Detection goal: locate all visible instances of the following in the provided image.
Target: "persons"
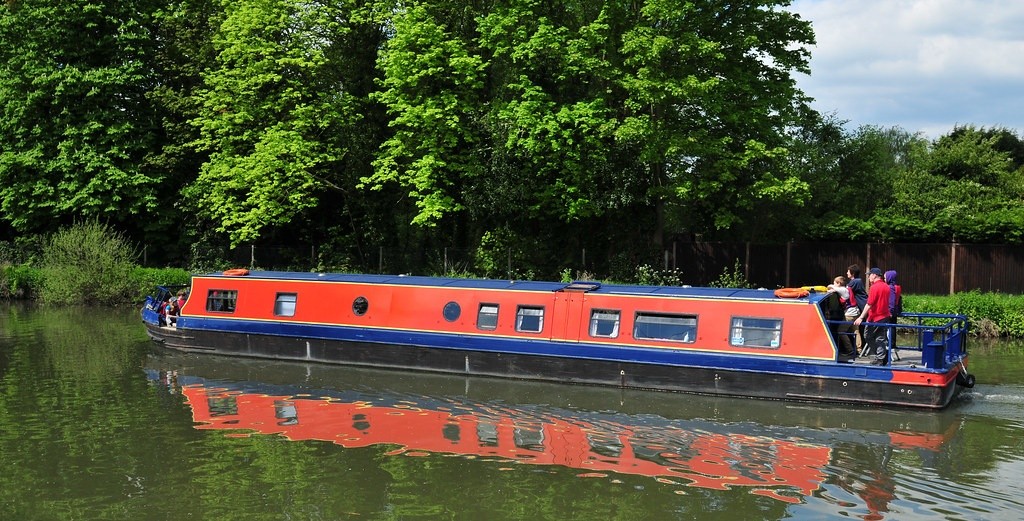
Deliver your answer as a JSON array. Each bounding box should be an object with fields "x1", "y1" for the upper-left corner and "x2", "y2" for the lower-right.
[
  {"x1": 827, "y1": 276, "x2": 862, "y2": 357},
  {"x1": 853, "y1": 268, "x2": 890, "y2": 366},
  {"x1": 884, "y1": 270, "x2": 900, "y2": 361},
  {"x1": 211, "y1": 292, "x2": 221, "y2": 311},
  {"x1": 176, "y1": 292, "x2": 186, "y2": 309},
  {"x1": 165, "y1": 297, "x2": 180, "y2": 327},
  {"x1": 846, "y1": 264, "x2": 868, "y2": 349}
]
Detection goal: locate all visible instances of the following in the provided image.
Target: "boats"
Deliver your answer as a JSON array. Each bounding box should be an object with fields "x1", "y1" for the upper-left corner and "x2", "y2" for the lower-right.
[
  {"x1": 140, "y1": 267, "x2": 977, "y2": 411},
  {"x1": 133, "y1": 342, "x2": 976, "y2": 507}
]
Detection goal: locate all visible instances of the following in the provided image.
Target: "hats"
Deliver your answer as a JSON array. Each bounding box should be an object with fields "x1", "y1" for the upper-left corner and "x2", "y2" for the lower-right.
[
  {"x1": 865, "y1": 268, "x2": 882, "y2": 276},
  {"x1": 169, "y1": 297, "x2": 177, "y2": 303}
]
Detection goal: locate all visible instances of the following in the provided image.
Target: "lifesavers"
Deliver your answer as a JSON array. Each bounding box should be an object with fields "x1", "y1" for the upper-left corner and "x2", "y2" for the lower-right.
[
  {"x1": 223, "y1": 268, "x2": 249, "y2": 276},
  {"x1": 774, "y1": 288, "x2": 809, "y2": 298}
]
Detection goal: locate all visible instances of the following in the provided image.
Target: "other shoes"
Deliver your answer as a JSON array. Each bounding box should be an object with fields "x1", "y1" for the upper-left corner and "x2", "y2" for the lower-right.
[
  {"x1": 870, "y1": 359, "x2": 886, "y2": 366},
  {"x1": 841, "y1": 351, "x2": 854, "y2": 356},
  {"x1": 856, "y1": 353, "x2": 860, "y2": 356}
]
